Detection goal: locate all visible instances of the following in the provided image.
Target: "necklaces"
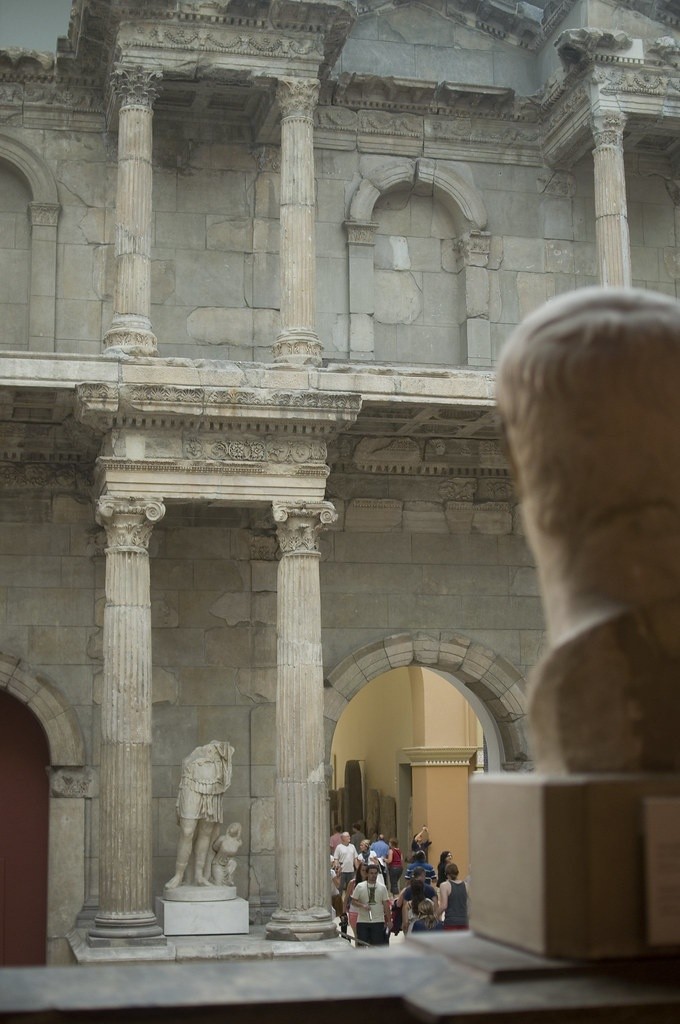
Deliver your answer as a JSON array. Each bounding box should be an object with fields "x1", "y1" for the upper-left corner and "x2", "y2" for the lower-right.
[{"x1": 367, "y1": 881, "x2": 376, "y2": 899}]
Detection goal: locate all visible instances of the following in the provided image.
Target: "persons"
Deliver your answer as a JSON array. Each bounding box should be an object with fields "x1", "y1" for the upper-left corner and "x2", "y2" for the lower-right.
[
  {"x1": 165, "y1": 740, "x2": 234, "y2": 890},
  {"x1": 329, "y1": 822, "x2": 471, "y2": 947},
  {"x1": 208, "y1": 822, "x2": 243, "y2": 887}
]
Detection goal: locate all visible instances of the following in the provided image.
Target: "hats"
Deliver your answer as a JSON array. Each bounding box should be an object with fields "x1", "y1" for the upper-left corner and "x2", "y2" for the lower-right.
[{"x1": 362, "y1": 840, "x2": 370, "y2": 847}]
